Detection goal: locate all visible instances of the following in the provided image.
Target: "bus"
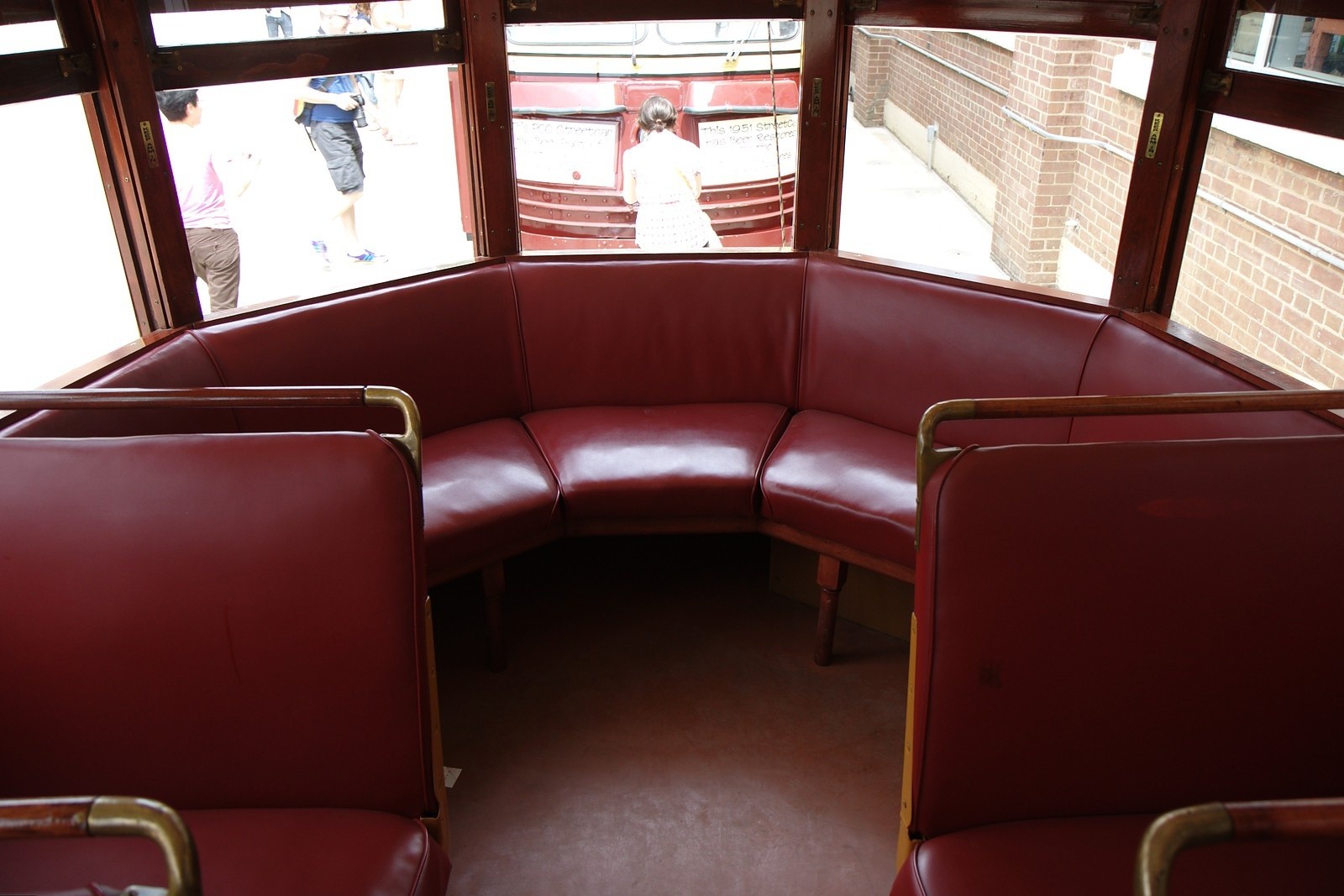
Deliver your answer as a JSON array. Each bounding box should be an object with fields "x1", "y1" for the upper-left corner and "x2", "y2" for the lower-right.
[{"x1": 449, "y1": 17, "x2": 803, "y2": 255}]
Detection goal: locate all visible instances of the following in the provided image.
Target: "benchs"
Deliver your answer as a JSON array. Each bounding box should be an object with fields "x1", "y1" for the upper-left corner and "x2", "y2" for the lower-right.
[{"x1": 0, "y1": 251, "x2": 1344, "y2": 896}]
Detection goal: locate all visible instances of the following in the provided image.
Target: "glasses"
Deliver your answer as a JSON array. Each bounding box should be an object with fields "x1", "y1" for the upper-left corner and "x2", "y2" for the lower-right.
[{"x1": 331, "y1": 13, "x2": 350, "y2": 21}]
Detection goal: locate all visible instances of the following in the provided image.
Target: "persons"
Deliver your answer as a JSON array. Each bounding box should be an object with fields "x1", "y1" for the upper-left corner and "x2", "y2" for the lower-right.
[
  {"x1": 623, "y1": 95, "x2": 711, "y2": 250},
  {"x1": 265, "y1": 7, "x2": 293, "y2": 39},
  {"x1": 350, "y1": 2, "x2": 412, "y2": 141},
  {"x1": 160, "y1": 90, "x2": 257, "y2": 312},
  {"x1": 282, "y1": 6, "x2": 388, "y2": 268}
]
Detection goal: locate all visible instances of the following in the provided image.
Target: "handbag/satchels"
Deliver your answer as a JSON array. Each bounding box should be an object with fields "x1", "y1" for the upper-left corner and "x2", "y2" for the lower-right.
[
  {"x1": 294, "y1": 98, "x2": 314, "y2": 124},
  {"x1": 703, "y1": 212, "x2": 722, "y2": 247}
]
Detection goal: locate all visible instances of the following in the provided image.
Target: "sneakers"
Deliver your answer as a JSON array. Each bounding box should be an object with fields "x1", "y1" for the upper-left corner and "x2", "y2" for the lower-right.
[
  {"x1": 309, "y1": 240, "x2": 335, "y2": 272},
  {"x1": 347, "y1": 250, "x2": 388, "y2": 264}
]
